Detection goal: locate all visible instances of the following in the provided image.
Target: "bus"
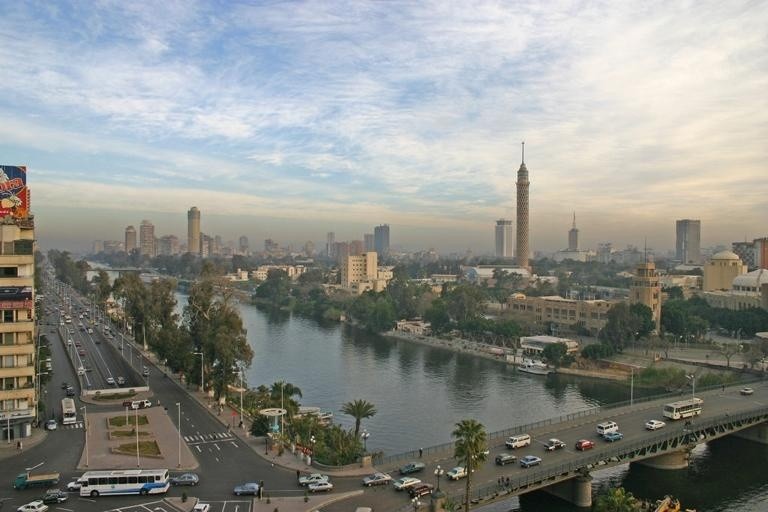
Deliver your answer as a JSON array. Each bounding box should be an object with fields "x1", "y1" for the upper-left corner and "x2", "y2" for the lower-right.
[
  {"x1": 663, "y1": 398, "x2": 704, "y2": 421},
  {"x1": 80, "y1": 469, "x2": 170, "y2": 498},
  {"x1": 61, "y1": 398, "x2": 77, "y2": 424}
]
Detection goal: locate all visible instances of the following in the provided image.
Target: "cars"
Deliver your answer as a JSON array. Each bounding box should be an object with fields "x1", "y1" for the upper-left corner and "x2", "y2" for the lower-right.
[
  {"x1": 740, "y1": 388, "x2": 754, "y2": 395},
  {"x1": 46, "y1": 420, "x2": 58, "y2": 431},
  {"x1": 17, "y1": 478, "x2": 81, "y2": 512},
  {"x1": 42, "y1": 281, "x2": 150, "y2": 396},
  {"x1": 299, "y1": 474, "x2": 333, "y2": 492},
  {"x1": 192, "y1": 503, "x2": 210, "y2": 512},
  {"x1": 234, "y1": 483, "x2": 260, "y2": 496},
  {"x1": 646, "y1": 420, "x2": 666, "y2": 431},
  {"x1": 172, "y1": 474, "x2": 199, "y2": 486},
  {"x1": 363, "y1": 421, "x2": 623, "y2": 498}
]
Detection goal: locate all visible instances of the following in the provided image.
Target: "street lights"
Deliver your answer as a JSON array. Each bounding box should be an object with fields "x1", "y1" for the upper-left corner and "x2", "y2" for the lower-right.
[
  {"x1": 631, "y1": 366, "x2": 641, "y2": 406},
  {"x1": 80, "y1": 402, "x2": 181, "y2": 467},
  {"x1": 685, "y1": 374, "x2": 694, "y2": 425},
  {"x1": 232, "y1": 371, "x2": 243, "y2": 421},
  {"x1": 412, "y1": 497, "x2": 421, "y2": 512},
  {"x1": 282, "y1": 382, "x2": 286, "y2": 436},
  {"x1": 361, "y1": 428, "x2": 370, "y2": 450},
  {"x1": 194, "y1": 353, "x2": 204, "y2": 392},
  {"x1": 310, "y1": 436, "x2": 315, "y2": 455}
]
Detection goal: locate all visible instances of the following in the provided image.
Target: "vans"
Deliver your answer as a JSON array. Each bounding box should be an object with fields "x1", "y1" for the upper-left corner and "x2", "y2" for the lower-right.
[
  {"x1": 131, "y1": 400, "x2": 152, "y2": 410},
  {"x1": 356, "y1": 507, "x2": 372, "y2": 512}
]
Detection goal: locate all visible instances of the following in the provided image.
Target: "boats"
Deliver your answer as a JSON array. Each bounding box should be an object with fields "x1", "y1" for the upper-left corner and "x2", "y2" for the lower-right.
[{"x1": 517, "y1": 363, "x2": 556, "y2": 375}]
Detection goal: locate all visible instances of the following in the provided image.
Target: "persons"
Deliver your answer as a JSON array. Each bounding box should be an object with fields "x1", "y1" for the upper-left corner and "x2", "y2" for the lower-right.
[
  {"x1": 296, "y1": 468, "x2": 300, "y2": 480},
  {"x1": 497, "y1": 475, "x2": 510, "y2": 487},
  {"x1": 419, "y1": 447, "x2": 424, "y2": 457}
]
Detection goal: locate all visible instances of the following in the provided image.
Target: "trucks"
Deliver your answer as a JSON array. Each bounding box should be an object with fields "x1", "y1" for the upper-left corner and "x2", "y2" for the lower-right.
[{"x1": 13, "y1": 472, "x2": 60, "y2": 491}]
